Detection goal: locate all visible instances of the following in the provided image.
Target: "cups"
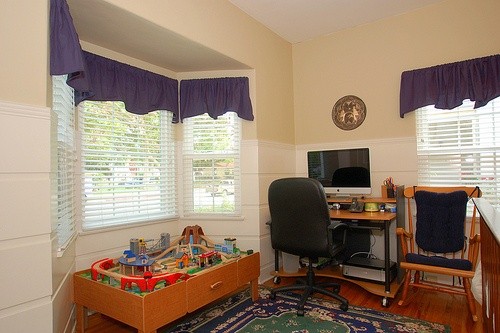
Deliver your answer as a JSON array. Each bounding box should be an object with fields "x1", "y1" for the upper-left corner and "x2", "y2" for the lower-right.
[{"x1": 387, "y1": 188, "x2": 394, "y2": 199}]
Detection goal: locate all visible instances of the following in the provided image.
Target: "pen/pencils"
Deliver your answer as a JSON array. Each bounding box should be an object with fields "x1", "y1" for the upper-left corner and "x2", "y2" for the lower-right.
[{"x1": 384, "y1": 177, "x2": 393, "y2": 189}]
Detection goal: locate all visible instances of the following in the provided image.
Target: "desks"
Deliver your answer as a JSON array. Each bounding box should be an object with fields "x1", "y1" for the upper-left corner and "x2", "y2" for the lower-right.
[
  {"x1": 71, "y1": 250, "x2": 260, "y2": 333},
  {"x1": 261, "y1": 189, "x2": 409, "y2": 307}
]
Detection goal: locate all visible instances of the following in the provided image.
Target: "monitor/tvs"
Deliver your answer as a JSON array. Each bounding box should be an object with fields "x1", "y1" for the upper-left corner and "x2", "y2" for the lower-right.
[{"x1": 307, "y1": 148, "x2": 372, "y2": 194}]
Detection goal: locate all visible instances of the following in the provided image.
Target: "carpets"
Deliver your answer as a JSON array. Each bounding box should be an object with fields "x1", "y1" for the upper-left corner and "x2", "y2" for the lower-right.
[{"x1": 158, "y1": 282, "x2": 452, "y2": 333}]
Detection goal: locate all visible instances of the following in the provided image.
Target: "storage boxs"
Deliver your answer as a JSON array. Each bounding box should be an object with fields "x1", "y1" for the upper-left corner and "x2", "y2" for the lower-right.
[{"x1": 343, "y1": 256, "x2": 397, "y2": 285}]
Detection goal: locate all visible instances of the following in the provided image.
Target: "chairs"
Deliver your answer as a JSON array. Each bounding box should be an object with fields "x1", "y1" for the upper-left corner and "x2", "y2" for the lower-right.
[
  {"x1": 396, "y1": 186, "x2": 484, "y2": 323},
  {"x1": 269, "y1": 178, "x2": 349, "y2": 317}
]
